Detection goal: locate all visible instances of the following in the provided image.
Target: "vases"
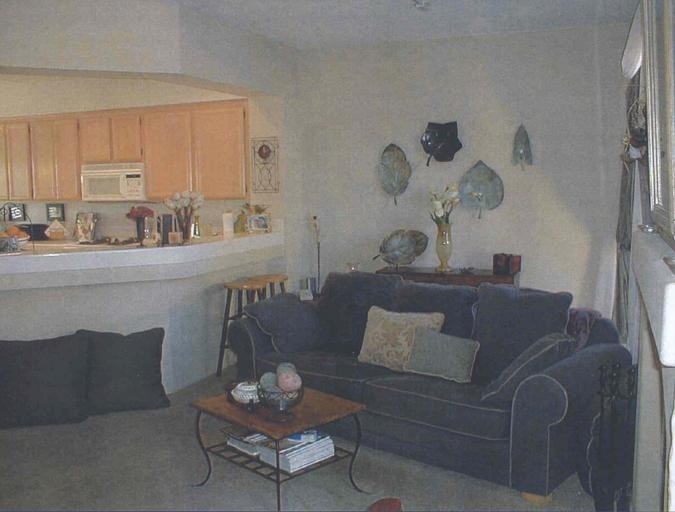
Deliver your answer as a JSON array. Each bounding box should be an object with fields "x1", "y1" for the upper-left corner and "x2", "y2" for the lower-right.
[
  {"x1": 136, "y1": 217, "x2": 145, "y2": 248},
  {"x1": 435, "y1": 221, "x2": 452, "y2": 273}
]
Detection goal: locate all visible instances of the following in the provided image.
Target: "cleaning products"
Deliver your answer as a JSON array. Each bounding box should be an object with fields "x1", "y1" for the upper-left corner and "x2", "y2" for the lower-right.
[{"x1": 193, "y1": 215, "x2": 202, "y2": 236}]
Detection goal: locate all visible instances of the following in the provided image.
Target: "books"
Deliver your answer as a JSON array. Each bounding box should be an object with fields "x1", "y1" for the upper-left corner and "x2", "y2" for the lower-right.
[{"x1": 219, "y1": 424, "x2": 336, "y2": 475}]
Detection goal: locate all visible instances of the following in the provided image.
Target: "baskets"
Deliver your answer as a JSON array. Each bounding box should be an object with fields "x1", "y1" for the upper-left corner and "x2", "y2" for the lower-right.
[{"x1": 257, "y1": 386, "x2": 305, "y2": 423}]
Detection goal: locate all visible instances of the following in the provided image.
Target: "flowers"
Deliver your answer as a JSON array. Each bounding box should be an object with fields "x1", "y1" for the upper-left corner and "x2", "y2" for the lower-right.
[
  {"x1": 420, "y1": 176, "x2": 485, "y2": 223},
  {"x1": 126, "y1": 205, "x2": 153, "y2": 219},
  {"x1": 163, "y1": 188, "x2": 203, "y2": 243}
]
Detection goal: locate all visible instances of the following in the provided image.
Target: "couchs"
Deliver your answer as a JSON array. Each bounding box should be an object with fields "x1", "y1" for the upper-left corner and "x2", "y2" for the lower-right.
[{"x1": 231, "y1": 274, "x2": 632, "y2": 495}]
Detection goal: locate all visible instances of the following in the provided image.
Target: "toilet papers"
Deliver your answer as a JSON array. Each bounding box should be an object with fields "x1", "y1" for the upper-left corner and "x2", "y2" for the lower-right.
[{"x1": 222, "y1": 213, "x2": 234, "y2": 234}]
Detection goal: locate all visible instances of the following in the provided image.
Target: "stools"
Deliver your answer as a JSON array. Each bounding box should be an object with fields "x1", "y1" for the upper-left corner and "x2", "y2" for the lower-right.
[
  {"x1": 216, "y1": 280, "x2": 267, "y2": 379},
  {"x1": 251, "y1": 263, "x2": 288, "y2": 297}
]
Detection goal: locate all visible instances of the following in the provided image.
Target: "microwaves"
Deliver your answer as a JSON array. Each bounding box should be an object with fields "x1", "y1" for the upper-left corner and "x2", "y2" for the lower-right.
[{"x1": 80, "y1": 163, "x2": 144, "y2": 202}]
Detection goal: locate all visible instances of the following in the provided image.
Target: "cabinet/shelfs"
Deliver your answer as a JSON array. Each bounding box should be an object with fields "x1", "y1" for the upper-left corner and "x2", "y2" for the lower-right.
[
  {"x1": 140, "y1": 97, "x2": 248, "y2": 202},
  {"x1": 0, "y1": 111, "x2": 33, "y2": 203},
  {"x1": 26, "y1": 112, "x2": 79, "y2": 202},
  {"x1": 73, "y1": 108, "x2": 143, "y2": 162}
]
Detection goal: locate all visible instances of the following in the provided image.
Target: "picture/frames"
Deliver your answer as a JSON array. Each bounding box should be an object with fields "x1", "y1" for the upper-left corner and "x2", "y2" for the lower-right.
[
  {"x1": 46, "y1": 203, "x2": 64, "y2": 221},
  {"x1": 8, "y1": 204, "x2": 24, "y2": 221},
  {"x1": 638, "y1": 0, "x2": 675, "y2": 251}
]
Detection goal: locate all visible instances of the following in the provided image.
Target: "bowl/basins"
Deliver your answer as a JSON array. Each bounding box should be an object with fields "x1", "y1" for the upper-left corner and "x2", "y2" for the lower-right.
[{"x1": 17, "y1": 234, "x2": 30, "y2": 248}]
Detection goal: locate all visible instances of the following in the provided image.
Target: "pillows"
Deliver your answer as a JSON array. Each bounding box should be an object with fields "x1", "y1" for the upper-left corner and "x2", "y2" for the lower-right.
[
  {"x1": 0, "y1": 330, "x2": 94, "y2": 425},
  {"x1": 354, "y1": 298, "x2": 447, "y2": 377},
  {"x1": 401, "y1": 322, "x2": 481, "y2": 380},
  {"x1": 77, "y1": 322, "x2": 174, "y2": 415},
  {"x1": 247, "y1": 294, "x2": 319, "y2": 356},
  {"x1": 480, "y1": 327, "x2": 578, "y2": 410}
]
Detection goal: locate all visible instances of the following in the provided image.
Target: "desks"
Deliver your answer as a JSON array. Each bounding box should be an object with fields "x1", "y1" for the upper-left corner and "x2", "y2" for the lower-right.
[{"x1": 373, "y1": 263, "x2": 520, "y2": 288}]
